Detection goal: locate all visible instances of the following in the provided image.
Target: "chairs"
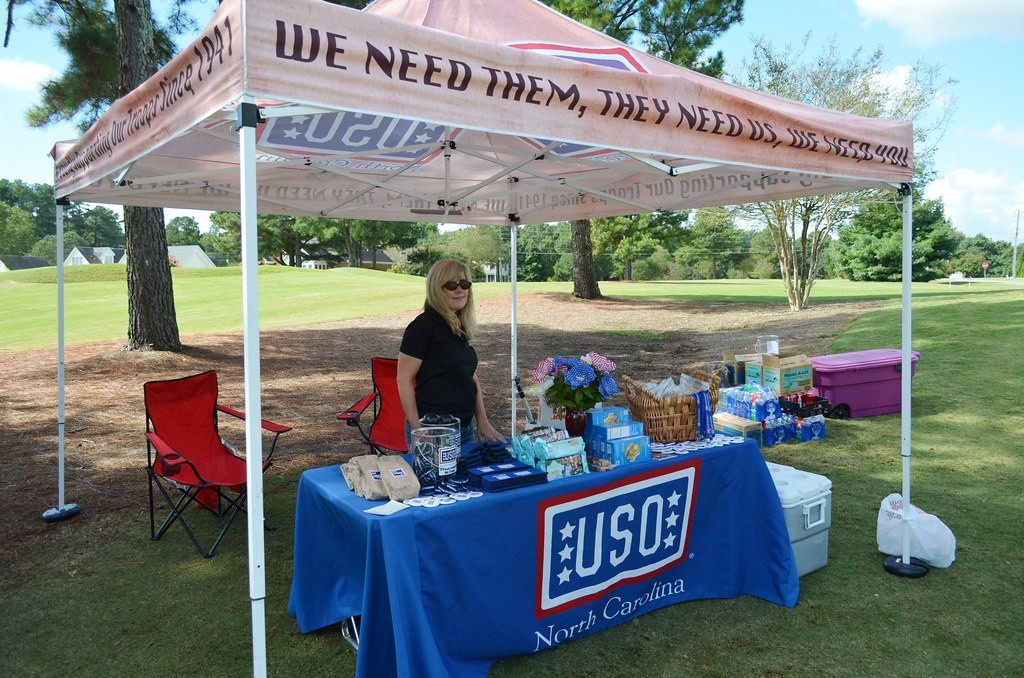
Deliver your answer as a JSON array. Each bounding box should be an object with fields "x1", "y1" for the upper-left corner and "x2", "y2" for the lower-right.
[
  {"x1": 336, "y1": 357, "x2": 415, "y2": 455},
  {"x1": 142, "y1": 369, "x2": 293, "y2": 559}
]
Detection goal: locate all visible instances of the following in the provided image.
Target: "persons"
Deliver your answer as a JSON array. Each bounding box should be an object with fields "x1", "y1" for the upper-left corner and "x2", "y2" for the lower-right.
[{"x1": 395, "y1": 258, "x2": 511, "y2": 457}]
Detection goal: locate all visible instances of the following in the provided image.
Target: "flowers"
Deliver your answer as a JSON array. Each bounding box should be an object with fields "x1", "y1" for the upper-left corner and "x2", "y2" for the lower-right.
[{"x1": 532, "y1": 351, "x2": 620, "y2": 420}]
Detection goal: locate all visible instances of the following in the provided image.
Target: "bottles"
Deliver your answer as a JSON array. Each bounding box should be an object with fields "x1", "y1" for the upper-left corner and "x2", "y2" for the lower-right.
[{"x1": 716, "y1": 384, "x2": 826, "y2": 447}]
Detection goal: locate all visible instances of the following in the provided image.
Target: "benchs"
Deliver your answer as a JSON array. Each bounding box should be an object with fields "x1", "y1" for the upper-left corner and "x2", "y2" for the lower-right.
[{"x1": 948, "y1": 271, "x2": 970, "y2": 285}]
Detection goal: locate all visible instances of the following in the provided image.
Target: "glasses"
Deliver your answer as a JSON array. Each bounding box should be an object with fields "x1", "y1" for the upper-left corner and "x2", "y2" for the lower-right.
[{"x1": 444, "y1": 279, "x2": 473, "y2": 291}]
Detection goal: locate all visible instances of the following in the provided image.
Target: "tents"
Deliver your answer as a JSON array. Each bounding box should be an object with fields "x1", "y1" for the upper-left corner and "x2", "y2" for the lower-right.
[{"x1": 39, "y1": 0, "x2": 929, "y2": 678}]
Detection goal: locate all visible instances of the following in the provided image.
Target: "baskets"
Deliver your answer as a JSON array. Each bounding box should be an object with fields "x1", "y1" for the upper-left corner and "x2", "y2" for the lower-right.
[{"x1": 623, "y1": 369, "x2": 718, "y2": 443}]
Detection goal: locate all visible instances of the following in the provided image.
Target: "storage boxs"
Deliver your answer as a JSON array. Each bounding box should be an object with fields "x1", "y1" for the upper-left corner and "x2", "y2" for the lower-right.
[
  {"x1": 537, "y1": 396, "x2": 651, "y2": 467},
  {"x1": 765, "y1": 461, "x2": 832, "y2": 581},
  {"x1": 706, "y1": 347, "x2": 922, "y2": 450}
]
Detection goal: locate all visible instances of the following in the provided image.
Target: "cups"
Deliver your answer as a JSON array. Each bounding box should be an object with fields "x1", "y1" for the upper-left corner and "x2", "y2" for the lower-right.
[
  {"x1": 410, "y1": 427, "x2": 457, "y2": 494},
  {"x1": 418, "y1": 417, "x2": 462, "y2": 468},
  {"x1": 754, "y1": 335, "x2": 779, "y2": 362}
]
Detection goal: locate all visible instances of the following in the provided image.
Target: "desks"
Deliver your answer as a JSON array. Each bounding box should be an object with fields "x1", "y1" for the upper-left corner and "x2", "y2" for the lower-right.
[{"x1": 284, "y1": 439, "x2": 800, "y2": 677}]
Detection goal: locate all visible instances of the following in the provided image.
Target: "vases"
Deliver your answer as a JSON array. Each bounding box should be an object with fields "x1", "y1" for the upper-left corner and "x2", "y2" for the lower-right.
[{"x1": 565, "y1": 410, "x2": 587, "y2": 438}]
